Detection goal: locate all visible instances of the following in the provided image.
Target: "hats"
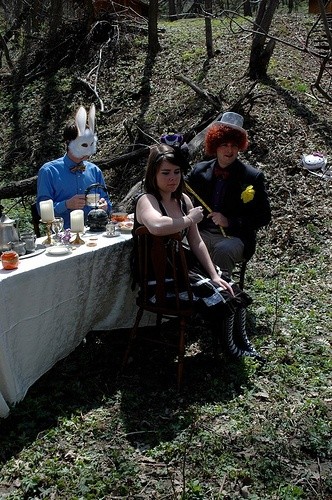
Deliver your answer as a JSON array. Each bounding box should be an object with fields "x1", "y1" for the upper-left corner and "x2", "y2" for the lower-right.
[{"x1": 211, "y1": 112, "x2": 246, "y2": 132}]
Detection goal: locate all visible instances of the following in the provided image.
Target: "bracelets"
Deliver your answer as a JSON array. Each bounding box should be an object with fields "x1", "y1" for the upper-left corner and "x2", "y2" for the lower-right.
[{"x1": 186, "y1": 216, "x2": 194, "y2": 226}]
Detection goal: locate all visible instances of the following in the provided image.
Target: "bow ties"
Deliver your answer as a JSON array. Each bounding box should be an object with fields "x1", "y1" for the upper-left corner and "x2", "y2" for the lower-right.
[
  {"x1": 214, "y1": 167, "x2": 230, "y2": 181},
  {"x1": 69, "y1": 164, "x2": 85, "y2": 175}
]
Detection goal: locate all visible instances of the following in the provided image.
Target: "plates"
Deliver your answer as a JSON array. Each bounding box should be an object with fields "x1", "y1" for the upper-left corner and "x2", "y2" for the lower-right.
[
  {"x1": 127, "y1": 213, "x2": 134, "y2": 221},
  {"x1": 103, "y1": 232, "x2": 120, "y2": 237},
  {"x1": 48, "y1": 247, "x2": 69, "y2": 255},
  {"x1": 115, "y1": 222, "x2": 134, "y2": 230}
]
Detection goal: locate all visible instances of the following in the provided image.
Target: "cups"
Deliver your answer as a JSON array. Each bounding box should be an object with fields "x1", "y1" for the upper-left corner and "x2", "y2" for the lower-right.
[
  {"x1": 106, "y1": 224, "x2": 115, "y2": 235},
  {"x1": 86, "y1": 193, "x2": 100, "y2": 209},
  {"x1": 0, "y1": 233, "x2": 36, "y2": 256}
]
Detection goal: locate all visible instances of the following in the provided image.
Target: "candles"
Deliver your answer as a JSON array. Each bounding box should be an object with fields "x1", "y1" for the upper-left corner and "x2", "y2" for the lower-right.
[
  {"x1": 70, "y1": 209, "x2": 85, "y2": 231},
  {"x1": 39, "y1": 198, "x2": 55, "y2": 222}
]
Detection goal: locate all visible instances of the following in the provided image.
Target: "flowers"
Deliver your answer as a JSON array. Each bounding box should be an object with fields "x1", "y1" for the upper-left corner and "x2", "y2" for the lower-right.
[{"x1": 240, "y1": 184, "x2": 255, "y2": 204}]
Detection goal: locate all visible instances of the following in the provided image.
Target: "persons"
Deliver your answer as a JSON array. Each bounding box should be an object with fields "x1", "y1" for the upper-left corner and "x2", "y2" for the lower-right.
[
  {"x1": 127, "y1": 143, "x2": 268, "y2": 363},
  {"x1": 183, "y1": 112, "x2": 270, "y2": 277},
  {"x1": 36, "y1": 123, "x2": 112, "y2": 232}
]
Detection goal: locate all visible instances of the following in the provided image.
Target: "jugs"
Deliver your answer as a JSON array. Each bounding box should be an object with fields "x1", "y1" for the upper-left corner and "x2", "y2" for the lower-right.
[{"x1": 0, "y1": 211, "x2": 19, "y2": 257}]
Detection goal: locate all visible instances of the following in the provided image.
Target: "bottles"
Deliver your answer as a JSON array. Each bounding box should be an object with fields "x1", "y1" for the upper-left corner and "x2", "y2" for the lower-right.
[
  {"x1": 87, "y1": 206, "x2": 108, "y2": 232},
  {"x1": 1, "y1": 251, "x2": 19, "y2": 270}
]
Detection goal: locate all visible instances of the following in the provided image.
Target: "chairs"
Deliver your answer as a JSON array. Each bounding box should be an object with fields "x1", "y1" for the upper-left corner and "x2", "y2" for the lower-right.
[{"x1": 133, "y1": 226, "x2": 218, "y2": 397}]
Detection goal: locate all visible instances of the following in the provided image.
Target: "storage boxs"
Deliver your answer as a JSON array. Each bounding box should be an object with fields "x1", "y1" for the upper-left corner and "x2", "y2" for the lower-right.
[{"x1": 111, "y1": 212, "x2": 128, "y2": 224}]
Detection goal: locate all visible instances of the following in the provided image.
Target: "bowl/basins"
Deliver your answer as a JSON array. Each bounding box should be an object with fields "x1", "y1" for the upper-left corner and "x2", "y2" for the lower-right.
[{"x1": 112, "y1": 213, "x2": 129, "y2": 221}]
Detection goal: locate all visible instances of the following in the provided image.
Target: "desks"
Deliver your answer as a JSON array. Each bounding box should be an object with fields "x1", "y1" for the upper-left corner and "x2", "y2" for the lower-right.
[{"x1": 0, "y1": 225, "x2": 189, "y2": 420}]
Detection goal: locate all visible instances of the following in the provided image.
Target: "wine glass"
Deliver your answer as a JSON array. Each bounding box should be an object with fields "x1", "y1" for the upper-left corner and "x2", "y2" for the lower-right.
[{"x1": 51, "y1": 216, "x2": 65, "y2": 244}]
